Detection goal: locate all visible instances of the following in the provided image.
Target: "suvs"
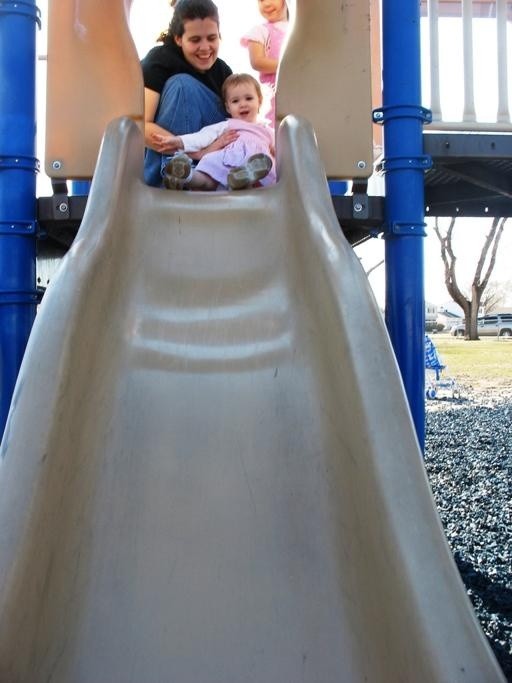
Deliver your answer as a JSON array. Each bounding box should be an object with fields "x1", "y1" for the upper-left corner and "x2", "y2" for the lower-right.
[{"x1": 450, "y1": 312, "x2": 512, "y2": 337}]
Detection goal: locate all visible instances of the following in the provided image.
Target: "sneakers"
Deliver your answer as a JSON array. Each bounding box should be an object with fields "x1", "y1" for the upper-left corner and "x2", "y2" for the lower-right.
[
  {"x1": 162, "y1": 155, "x2": 191, "y2": 190},
  {"x1": 228, "y1": 153, "x2": 272, "y2": 188}
]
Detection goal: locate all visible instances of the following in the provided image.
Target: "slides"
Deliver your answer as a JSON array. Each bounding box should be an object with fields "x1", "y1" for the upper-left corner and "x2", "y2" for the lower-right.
[{"x1": 0, "y1": 115, "x2": 507, "y2": 681}]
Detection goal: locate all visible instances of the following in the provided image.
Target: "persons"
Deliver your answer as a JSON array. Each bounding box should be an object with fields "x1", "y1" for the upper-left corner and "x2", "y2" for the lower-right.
[
  {"x1": 151, "y1": 73, "x2": 277, "y2": 190},
  {"x1": 239, "y1": 0, "x2": 291, "y2": 86},
  {"x1": 140, "y1": 1, "x2": 233, "y2": 188}
]
